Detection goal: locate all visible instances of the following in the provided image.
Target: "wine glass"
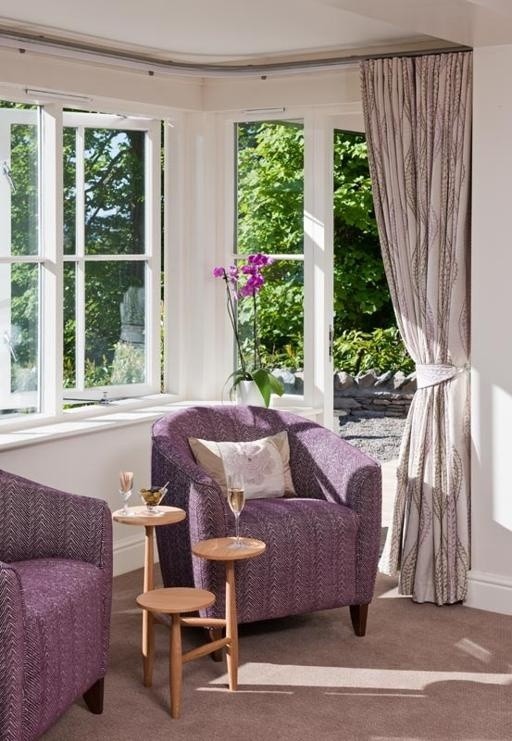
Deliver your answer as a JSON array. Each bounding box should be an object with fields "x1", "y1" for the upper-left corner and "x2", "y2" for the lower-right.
[
  {"x1": 228, "y1": 488, "x2": 245, "y2": 549},
  {"x1": 118, "y1": 472, "x2": 167, "y2": 517}
]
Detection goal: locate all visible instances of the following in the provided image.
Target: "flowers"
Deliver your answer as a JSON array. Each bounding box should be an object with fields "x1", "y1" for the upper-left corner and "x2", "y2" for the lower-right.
[{"x1": 214, "y1": 253, "x2": 286, "y2": 409}]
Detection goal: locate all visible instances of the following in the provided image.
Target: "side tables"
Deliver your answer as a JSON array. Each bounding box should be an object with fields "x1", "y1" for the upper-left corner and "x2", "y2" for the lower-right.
[{"x1": 110, "y1": 504, "x2": 266, "y2": 719}]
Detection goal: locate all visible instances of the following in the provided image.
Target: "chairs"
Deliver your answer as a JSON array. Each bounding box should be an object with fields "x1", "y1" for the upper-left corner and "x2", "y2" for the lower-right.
[
  {"x1": 151, "y1": 404, "x2": 382, "y2": 663},
  {"x1": 0, "y1": 404, "x2": 114, "y2": 740}
]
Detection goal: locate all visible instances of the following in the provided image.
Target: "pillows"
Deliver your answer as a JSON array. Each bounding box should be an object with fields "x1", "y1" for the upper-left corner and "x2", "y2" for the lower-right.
[{"x1": 187, "y1": 431, "x2": 299, "y2": 500}]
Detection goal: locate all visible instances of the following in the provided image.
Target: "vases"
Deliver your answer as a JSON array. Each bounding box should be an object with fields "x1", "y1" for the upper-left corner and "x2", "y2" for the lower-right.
[{"x1": 237, "y1": 380, "x2": 267, "y2": 407}]
